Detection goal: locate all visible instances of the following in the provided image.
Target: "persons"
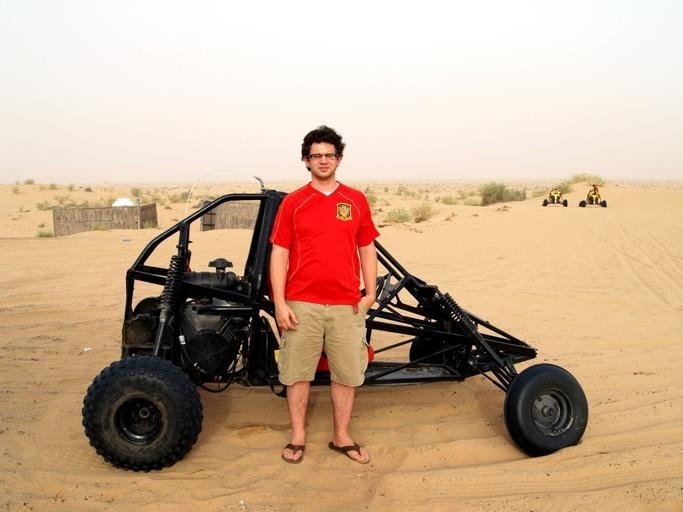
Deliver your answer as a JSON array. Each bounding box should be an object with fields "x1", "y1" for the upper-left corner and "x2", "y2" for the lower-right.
[
  {"x1": 592, "y1": 185, "x2": 598, "y2": 194},
  {"x1": 268, "y1": 126, "x2": 380, "y2": 464}
]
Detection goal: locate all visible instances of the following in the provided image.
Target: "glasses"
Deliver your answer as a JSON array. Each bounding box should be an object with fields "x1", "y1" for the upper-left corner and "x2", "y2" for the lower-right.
[{"x1": 307, "y1": 153, "x2": 339, "y2": 161}]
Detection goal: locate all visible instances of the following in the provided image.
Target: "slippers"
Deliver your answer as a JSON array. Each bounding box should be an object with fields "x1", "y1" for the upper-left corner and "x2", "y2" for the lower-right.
[
  {"x1": 327, "y1": 441, "x2": 371, "y2": 465},
  {"x1": 282, "y1": 442, "x2": 305, "y2": 464}
]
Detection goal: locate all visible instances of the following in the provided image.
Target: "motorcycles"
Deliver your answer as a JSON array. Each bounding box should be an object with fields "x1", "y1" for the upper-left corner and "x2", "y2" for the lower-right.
[
  {"x1": 579, "y1": 185, "x2": 608, "y2": 208},
  {"x1": 541, "y1": 186, "x2": 569, "y2": 207},
  {"x1": 81, "y1": 179, "x2": 588, "y2": 472}
]
